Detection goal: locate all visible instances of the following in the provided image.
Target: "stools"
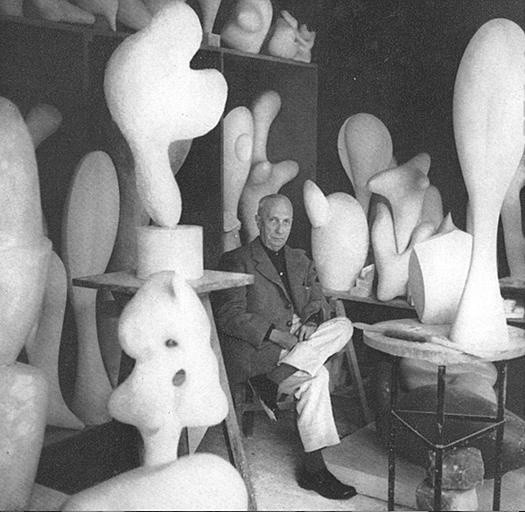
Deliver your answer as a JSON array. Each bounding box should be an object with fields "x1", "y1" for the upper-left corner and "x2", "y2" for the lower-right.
[{"x1": 234, "y1": 364, "x2": 303, "y2": 441}]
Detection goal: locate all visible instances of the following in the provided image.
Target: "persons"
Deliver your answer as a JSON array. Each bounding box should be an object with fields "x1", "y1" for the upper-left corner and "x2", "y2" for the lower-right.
[{"x1": 209, "y1": 194, "x2": 357, "y2": 500}]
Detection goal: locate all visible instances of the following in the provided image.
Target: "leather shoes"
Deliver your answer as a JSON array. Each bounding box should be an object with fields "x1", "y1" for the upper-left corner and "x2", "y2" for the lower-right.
[
  {"x1": 247, "y1": 373, "x2": 278, "y2": 422},
  {"x1": 296, "y1": 467, "x2": 357, "y2": 499}
]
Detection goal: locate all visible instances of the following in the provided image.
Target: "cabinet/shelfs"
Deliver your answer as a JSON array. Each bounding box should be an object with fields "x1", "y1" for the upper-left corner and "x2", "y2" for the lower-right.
[{"x1": 1, "y1": 14, "x2": 320, "y2": 463}]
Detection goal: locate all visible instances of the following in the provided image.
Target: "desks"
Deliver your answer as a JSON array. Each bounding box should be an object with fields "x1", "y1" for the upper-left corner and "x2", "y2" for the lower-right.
[
  {"x1": 69, "y1": 263, "x2": 258, "y2": 512},
  {"x1": 358, "y1": 313, "x2": 524, "y2": 511},
  {"x1": 317, "y1": 286, "x2": 525, "y2": 437}
]
